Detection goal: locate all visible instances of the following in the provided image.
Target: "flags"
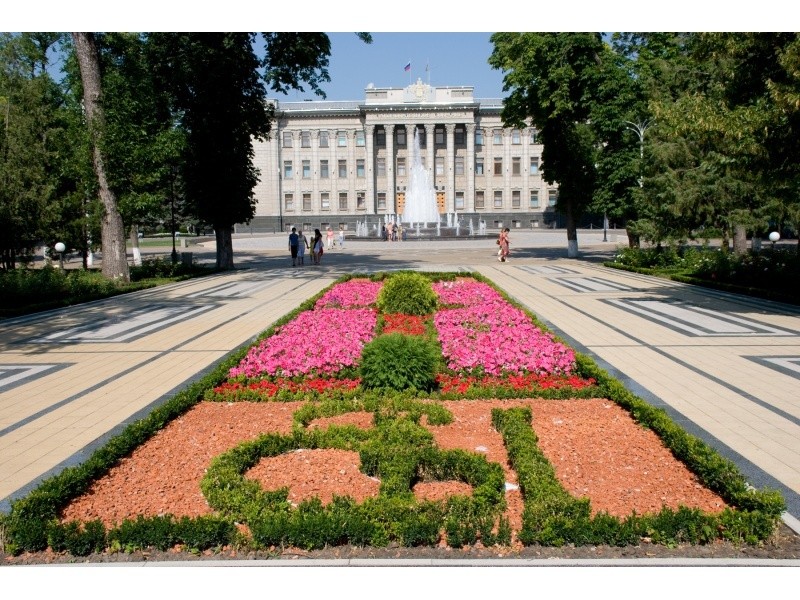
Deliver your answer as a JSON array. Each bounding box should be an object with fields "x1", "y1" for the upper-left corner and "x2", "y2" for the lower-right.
[{"x1": 404, "y1": 63, "x2": 410, "y2": 71}]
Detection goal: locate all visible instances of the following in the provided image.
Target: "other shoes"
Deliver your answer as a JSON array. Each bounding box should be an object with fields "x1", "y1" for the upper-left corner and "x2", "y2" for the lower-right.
[
  {"x1": 501, "y1": 257, "x2": 505, "y2": 262},
  {"x1": 498, "y1": 257, "x2": 501, "y2": 262}
]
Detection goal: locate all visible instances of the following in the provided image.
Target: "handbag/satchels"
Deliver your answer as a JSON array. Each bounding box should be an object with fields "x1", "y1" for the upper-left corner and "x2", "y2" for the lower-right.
[{"x1": 496, "y1": 240, "x2": 500, "y2": 245}]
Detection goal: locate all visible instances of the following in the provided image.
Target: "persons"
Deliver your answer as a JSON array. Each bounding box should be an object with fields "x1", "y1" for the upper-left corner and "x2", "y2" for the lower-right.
[
  {"x1": 382, "y1": 222, "x2": 402, "y2": 242},
  {"x1": 313, "y1": 229, "x2": 323, "y2": 265},
  {"x1": 308, "y1": 237, "x2": 315, "y2": 261},
  {"x1": 498, "y1": 227, "x2": 512, "y2": 262},
  {"x1": 326, "y1": 228, "x2": 334, "y2": 250},
  {"x1": 297, "y1": 230, "x2": 309, "y2": 265},
  {"x1": 340, "y1": 227, "x2": 344, "y2": 246},
  {"x1": 289, "y1": 227, "x2": 299, "y2": 266}
]
